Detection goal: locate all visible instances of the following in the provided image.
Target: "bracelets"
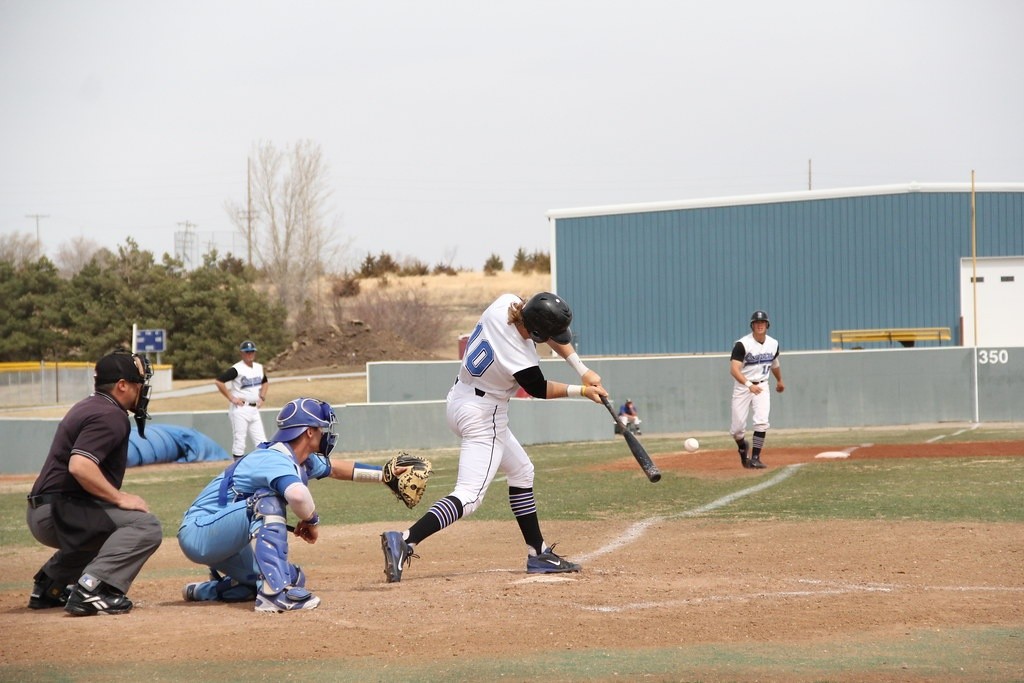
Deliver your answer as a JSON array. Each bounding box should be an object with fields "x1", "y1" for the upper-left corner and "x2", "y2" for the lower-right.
[
  {"x1": 745, "y1": 380, "x2": 753, "y2": 387},
  {"x1": 260, "y1": 396, "x2": 265, "y2": 401}
]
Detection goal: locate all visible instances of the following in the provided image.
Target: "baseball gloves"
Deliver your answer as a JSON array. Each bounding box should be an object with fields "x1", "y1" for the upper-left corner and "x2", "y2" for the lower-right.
[{"x1": 383, "y1": 451, "x2": 436, "y2": 510}]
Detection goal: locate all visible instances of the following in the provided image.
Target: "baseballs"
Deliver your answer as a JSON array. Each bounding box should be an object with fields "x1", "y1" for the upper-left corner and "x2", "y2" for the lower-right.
[{"x1": 683, "y1": 438, "x2": 699, "y2": 453}]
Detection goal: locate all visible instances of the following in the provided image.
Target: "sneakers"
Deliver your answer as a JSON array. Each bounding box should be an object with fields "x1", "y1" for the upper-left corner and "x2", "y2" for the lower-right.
[
  {"x1": 381, "y1": 529, "x2": 414, "y2": 584},
  {"x1": 65, "y1": 585, "x2": 134, "y2": 617},
  {"x1": 739, "y1": 441, "x2": 767, "y2": 468},
  {"x1": 527, "y1": 547, "x2": 582, "y2": 573},
  {"x1": 28, "y1": 583, "x2": 72, "y2": 610},
  {"x1": 181, "y1": 581, "x2": 207, "y2": 602},
  {"x1": 255, "y1": 588, "x2": 322, "y2": 613}
]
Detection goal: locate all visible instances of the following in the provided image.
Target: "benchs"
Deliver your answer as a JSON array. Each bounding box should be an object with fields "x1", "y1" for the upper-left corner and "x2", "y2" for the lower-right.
[{"x1": 831, "y1": 327, "x2": 952, "y2": 351}]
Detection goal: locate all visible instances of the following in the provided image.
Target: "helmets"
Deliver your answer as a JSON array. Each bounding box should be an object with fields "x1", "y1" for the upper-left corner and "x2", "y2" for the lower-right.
[
  {"x1": 750, "y1": 311, "x2": 770, "y2": 329},
  {"x1": 521, "y1": 291, "x2": 574, "y2": 345},
  {"x1": 268, "y1": 396, "x2": 333, "y2": 442}
]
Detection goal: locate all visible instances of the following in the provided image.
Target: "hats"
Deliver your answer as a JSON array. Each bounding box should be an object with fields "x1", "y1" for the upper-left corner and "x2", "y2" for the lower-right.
[
  {"x1": 94, "y1": 351, "x2": 149, "y2": 384},
  {"x1": 240, "y1": 340, "x2": 258, "y2": 352}
]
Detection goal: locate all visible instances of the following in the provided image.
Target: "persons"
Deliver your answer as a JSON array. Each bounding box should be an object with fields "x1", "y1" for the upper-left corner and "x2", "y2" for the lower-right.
[
  {"x1": 176, "y1": 395, "x2": 432, "y2": 613},
  {"x1": 618, "y1": 398, "x2": 642, "y2": 436},
  {"x1": 730, "y1": 310, "x2": 784, "y2": 470},
  {"x1": 380, "y1": 292, "x2": 608, "y2": 582},
  {"x1": 216, "y1": 341, "x2": 267, "y2": 462},
  {"x1": 25, "y1": 349, "x2": 161, "y2": 617}
]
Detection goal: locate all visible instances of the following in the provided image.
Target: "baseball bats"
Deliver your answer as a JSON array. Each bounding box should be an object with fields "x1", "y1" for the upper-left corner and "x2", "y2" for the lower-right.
[{"x1": 593, "y1": 384, "x2": 662, "y2": 483}]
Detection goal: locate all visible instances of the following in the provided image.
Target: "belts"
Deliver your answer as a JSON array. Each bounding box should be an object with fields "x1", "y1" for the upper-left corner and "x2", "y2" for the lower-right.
[
  {"x1": 752, "y1": 381, "x2": 765, "y2": 385},
  {"x1": 240, "y1": 402, "x2": 259, "y2": 407},
  {"x1": 28, "y1": 495, "x2": 49, "y2": 506},
  {"x1": 455, "y1": 378, "x2": 486, "y2": 397}
]
